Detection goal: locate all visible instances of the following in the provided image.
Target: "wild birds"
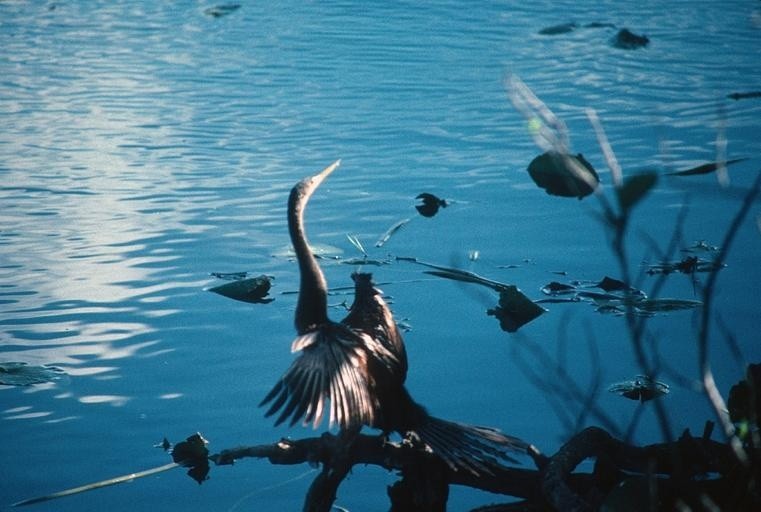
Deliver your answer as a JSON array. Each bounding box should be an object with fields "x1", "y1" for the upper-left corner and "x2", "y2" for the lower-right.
[{"x1": 256, "y1": 158, "x2": 533, "y2": 480}]
